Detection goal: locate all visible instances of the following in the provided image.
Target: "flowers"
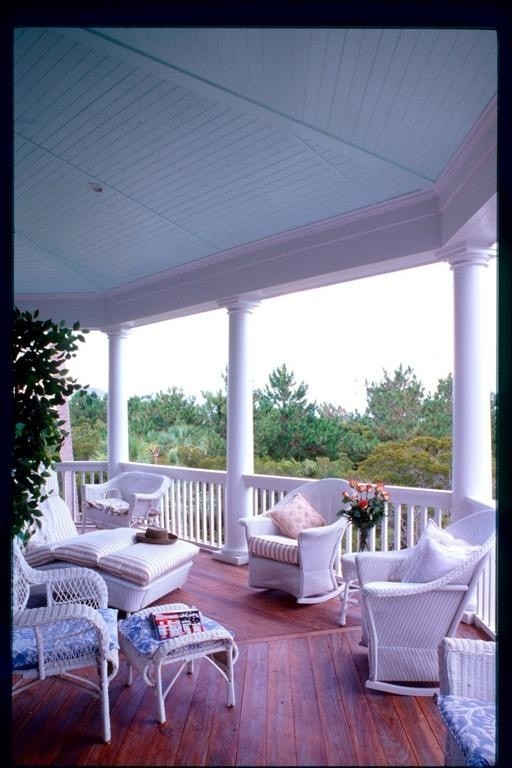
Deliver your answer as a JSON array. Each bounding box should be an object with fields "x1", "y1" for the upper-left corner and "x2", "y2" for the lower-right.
[{"x1": 342, "y1": 479, "x2": 390, "y2": 526}]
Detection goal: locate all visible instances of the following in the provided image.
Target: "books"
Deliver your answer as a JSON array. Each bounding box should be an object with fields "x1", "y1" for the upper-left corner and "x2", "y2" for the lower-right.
[{"x1": 152, "y1": 609, "x2": 206, "y2": 640}]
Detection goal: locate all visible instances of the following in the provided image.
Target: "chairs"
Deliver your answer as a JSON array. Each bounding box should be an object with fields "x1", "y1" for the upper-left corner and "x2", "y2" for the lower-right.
[
  {"x1": 75, "y1": 468, "x2": 172, "y2": 528},
  {"x1": 17, "y1": 494, "x2": 201, "y2": 614},
  {"x1": 237, "y1": 475, "x2": 348, "y2": 607},
  {"x1": 432, "y1": 630, "x2": 501, "y2": 767},
  {"x1": 9, "y1": 535, "x2": 135, "y2": 743},
  {"x1": 351, "y1": 509, "x2": 496, "y2": 698}
]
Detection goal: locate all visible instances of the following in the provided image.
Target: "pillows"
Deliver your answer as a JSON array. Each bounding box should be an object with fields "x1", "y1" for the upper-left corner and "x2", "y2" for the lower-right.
[{"x1": 266, "y1": 491, "x2": 323, "y2": 539}]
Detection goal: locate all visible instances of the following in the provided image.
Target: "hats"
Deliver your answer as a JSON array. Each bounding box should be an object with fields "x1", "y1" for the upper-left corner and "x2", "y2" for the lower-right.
[{"x1": 136, "y1": 526, "x2": 178, "y2": 544}]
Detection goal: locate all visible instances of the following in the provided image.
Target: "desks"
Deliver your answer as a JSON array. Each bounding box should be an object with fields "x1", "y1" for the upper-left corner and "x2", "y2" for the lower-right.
[
  {"x1": 340, "y1": 550, "x2": 357, "y2": 627},
  {"x1": 117, "y1": 600, "x2": 241, "y2": 726}
]
